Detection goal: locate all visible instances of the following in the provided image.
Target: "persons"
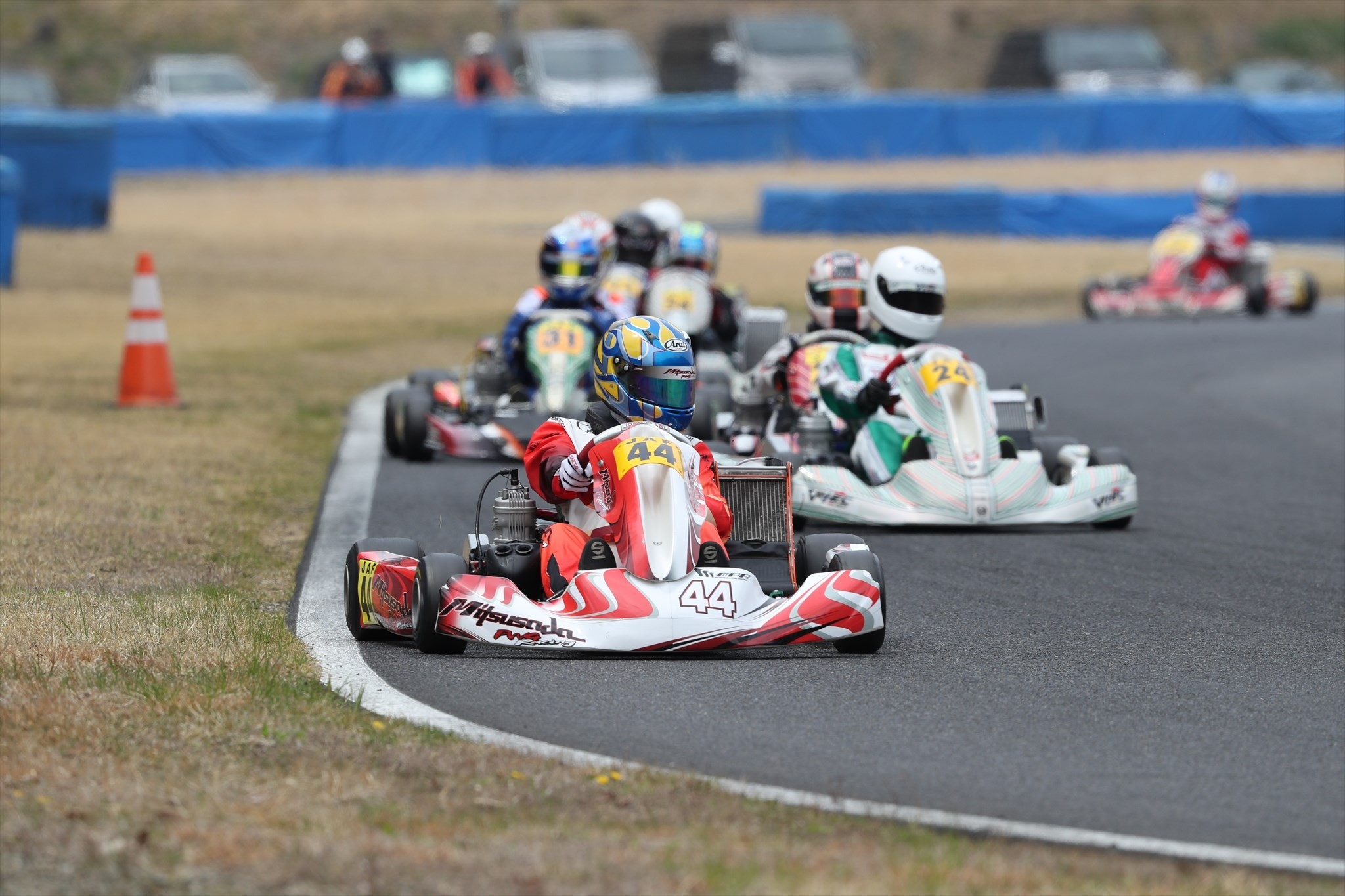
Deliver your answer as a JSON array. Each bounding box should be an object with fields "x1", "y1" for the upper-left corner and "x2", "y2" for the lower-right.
[
  {"x1": 455, "y1": 32, "x2": 515, "y2": 98},
  {"x1": 815, "y1": 246, "x2": 1020, "y2": 487},
  {"x1": 318, "y1": 38, "x2": 381, "y2": 99},
  {"x1": 501, "y1": 199, "x2": 876, "y2": 436},
  {"x1": 1169, "y1": 163, "x2": 1251, "y2": 286},
  {"x1": 523, "y1": 312, "x2": 733, "y2": 598}
]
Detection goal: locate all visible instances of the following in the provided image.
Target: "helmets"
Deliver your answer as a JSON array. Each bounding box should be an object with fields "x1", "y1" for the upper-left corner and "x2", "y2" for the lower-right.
[
  {"x1": 866, "y1": 247, "x2": 946, "y2": 342},
  {"x1": 805, "y1": 250, "x2": 872, "y2": 332},
  {"x1": 537, "y1": 225, "x2": 602, "y2": 300},
  {"x1": 637, "y1": 198, "x2": 684, "y2": 235},
  {"x1": 1194, "y1": 171, "x2": 1237, "y2": 219},
  {"x1": 641, "y1": 268, "x2": 712, "y2": 332},
  {"x1": 560, "y1": 213, "x2": 618, "y2": 281},
  {"x1": 613, "y1": 211, "x2": 660, "y2": 264},
  {"x1": 655, "y1": 221, "x2": 720, "y2": 278},
  {"x1": 594, "y1": 315, "x2": 695, "y2": 431}
]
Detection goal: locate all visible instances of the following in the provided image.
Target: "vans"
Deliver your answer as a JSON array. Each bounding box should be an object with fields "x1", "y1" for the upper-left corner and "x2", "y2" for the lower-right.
[{"x1": 657, "y1": 9, "x2": 864, "y2": 100}]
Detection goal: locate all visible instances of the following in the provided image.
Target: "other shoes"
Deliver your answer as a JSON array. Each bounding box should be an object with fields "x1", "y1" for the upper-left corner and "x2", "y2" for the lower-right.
[
  {"x1": 999, "y1": 434, "x2": 1018, "y2": 459},
  {"x1": 577, "y1": 538, "x2": 617, "y2": 571},
  {"x1": 900, "y1": 434, "x2": 930, "y2": 464},
  {"x1": 697, "y1": 541, "x2": 732, "y2": 567}
]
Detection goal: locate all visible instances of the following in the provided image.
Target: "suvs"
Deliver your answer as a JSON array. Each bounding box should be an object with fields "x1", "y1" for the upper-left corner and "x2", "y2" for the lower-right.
[
  {"x1": 501, "y1": 25, "x2": 660, "y2": 106},
  {"x1": 980, "y1": 20, "x2": 1200, "y2": 95}
]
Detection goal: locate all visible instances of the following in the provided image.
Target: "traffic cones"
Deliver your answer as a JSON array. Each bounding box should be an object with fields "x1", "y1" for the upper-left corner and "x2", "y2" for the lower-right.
[{"x1": 107, "y1": 251, "x2": 189, "y2": 411}]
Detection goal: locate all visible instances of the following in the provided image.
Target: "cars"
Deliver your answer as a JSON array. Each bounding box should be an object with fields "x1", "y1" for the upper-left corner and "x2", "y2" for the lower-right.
[
  {"x1": 305, "y1": 52, "x2": 459, "y2": 102},
  {"x1": 122, "y1": 53, "x2": 278, "y2": 112},
  {"x1": 1214, "y1": 51, "x2": 1336, "y2": 95},
  {"x1": 0, "y1": 68, "x2": 58, "y2": 108}
]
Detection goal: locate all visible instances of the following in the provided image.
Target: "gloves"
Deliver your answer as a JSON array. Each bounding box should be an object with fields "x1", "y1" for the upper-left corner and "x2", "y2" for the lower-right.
[
  {"x1": 858, "y1": 380, "x2": 894, "y2": 412},
  {"x1": 559, "y1": 453, "x2": 593, "y2": 492}
]
